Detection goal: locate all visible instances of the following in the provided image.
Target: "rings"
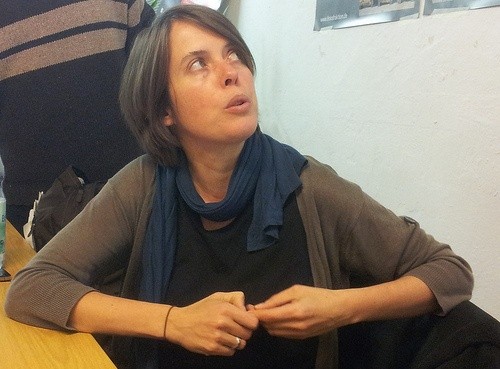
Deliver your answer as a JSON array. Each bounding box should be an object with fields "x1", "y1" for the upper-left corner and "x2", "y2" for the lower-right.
[{"x1": 230, "y1": 337, "x2": 241, "y2": 350}]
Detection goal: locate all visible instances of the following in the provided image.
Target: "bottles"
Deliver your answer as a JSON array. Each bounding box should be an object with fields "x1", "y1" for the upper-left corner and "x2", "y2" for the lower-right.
[{"x1": 0, "y1": 157, "x2": 7, "y2": 277}]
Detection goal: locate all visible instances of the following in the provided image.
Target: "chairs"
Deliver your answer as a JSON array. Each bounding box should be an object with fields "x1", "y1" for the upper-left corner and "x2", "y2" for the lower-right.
[{"x1": 343, "y1": 273, "x2": 500, "y2": 369}]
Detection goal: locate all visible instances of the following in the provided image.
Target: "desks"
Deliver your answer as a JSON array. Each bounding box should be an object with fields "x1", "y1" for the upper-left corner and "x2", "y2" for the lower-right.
[{"x1": 0, "y1": 220, "x2": 118, "y2": 369}]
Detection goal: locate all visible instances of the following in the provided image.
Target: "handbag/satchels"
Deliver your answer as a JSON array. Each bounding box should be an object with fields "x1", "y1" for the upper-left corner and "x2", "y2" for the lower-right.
[{"x1": 28, "y1": 165, "x2": 99, "y2": 251}]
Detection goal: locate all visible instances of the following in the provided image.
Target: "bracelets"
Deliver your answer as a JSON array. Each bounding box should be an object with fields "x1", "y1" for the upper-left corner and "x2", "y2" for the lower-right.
[{"x1": 163, "y1": 305, "x2": 175, "y2": 342}]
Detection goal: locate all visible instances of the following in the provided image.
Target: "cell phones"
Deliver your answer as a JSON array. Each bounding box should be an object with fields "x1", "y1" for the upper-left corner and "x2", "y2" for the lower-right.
[{"x1": 0, "y1": 268, "x2": 11, "y2": 281}]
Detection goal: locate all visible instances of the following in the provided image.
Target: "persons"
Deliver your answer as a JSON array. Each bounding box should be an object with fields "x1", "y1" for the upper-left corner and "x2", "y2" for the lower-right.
[
  {"x1": 0, "y1": 0, "x2": 159, "y2": 242},
  {"x1": 5, "y1": 4, "x2": 474, "y2": 369}
]
